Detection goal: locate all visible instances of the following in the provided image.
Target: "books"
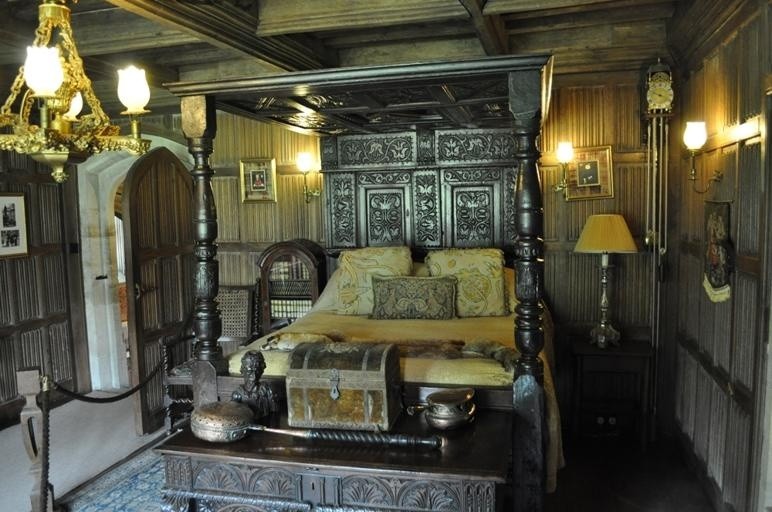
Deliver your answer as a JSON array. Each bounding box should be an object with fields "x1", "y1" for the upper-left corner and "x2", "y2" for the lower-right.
[
  {"x1": 270, "y1": 255, "x2": 311, "y2": 280},
  {"x1": 270, "y1": 299, "x2": 313, "y2": 318}
]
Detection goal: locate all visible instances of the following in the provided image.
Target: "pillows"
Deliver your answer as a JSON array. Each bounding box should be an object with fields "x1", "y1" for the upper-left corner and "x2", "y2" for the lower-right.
[
  {"x1": 425, "y1": 248, "x2": 507, "y2": 316},
  {"x1": 337, "y1": 246, "x2": 414, "y2": 316},
  {"x1": 370, "y1": 274, "x2": 458, "y2": 320}
]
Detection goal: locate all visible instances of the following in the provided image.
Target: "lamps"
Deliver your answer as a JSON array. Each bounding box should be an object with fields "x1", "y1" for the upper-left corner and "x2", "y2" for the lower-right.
[
  {"x1": 0, "y1": 0, "x2": 152, "y2": 183},
  {"x1": 296, "y1": 152, "x2": 321, "y2": 203},
  {"x1": 573, "y1": 214, "x2": 638, "y2": 349},
  {"x1": 683, "y1": 121, "x2": 723, "y2": 193},
  {"x1": 551, "y1": 142, "x2": 574, "y2": 192}
]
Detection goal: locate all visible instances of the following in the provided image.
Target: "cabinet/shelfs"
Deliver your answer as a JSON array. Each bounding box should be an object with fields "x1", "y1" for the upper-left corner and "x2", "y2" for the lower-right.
[{"x1": 257, "y1": 239, "x2": 327, "y2": 335}]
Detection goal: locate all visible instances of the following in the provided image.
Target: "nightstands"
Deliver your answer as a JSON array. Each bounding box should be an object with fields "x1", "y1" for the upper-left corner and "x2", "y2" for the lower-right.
[{"x1": 568, "y1": 335, "x2": 652, "y2": 454}]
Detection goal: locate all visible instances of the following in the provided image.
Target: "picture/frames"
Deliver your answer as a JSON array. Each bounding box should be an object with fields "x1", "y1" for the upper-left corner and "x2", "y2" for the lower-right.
[
  {"x1": 564, "y1": 145, "x2": 615, "y2": 202},
  {"x1": 0, "y1": 191, "x2": 31, "y2": 261},
  {"x1": 239, "y1": 157, "x2": 278, "y2": 203}
]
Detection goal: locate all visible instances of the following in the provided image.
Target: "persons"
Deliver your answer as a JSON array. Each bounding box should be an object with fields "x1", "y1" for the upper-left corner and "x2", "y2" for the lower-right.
[
  {"x1": 254, "y1": 175, "x2": 264, "y2": 186},
  {"x1": 232, "y1": 349, "x2": 280, "y2": 419}
]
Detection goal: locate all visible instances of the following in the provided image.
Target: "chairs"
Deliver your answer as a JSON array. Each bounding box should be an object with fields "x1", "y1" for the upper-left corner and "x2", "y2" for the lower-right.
[{"x1": 159, "y1": 278, "x2": 261, "y2": 437}]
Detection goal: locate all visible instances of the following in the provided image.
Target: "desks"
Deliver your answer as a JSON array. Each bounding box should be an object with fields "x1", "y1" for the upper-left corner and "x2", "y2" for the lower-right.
[{"x1": 153, "y1": 398, "x2": 515, "y2": 512}]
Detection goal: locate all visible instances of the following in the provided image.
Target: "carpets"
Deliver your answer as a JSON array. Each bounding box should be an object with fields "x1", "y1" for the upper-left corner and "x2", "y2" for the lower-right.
[{"x1": 53, "y1": 419, "x2": 190, "y2": 512}]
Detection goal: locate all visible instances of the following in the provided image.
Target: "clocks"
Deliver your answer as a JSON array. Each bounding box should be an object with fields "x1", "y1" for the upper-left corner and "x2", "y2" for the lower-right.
[{"x1": 640, "y1": 57, "x2": 675, "y2": 122}]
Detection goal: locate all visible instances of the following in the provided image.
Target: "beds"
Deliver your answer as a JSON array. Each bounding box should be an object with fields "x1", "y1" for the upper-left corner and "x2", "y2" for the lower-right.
[{"x1": 162, "y1": 46, "x2": 567, "y2": 512}]
